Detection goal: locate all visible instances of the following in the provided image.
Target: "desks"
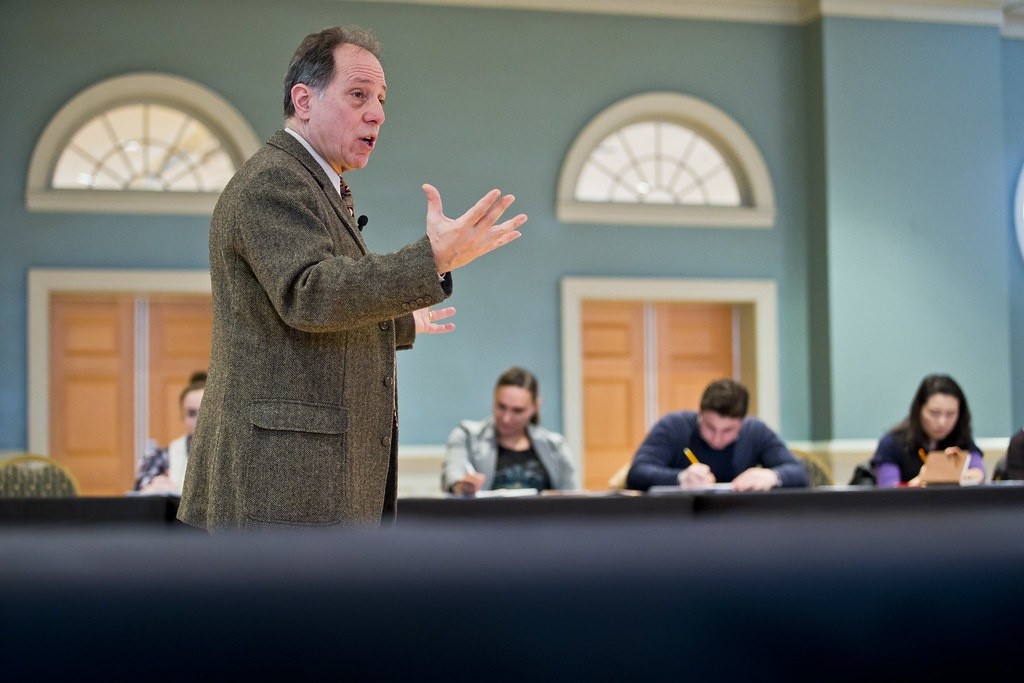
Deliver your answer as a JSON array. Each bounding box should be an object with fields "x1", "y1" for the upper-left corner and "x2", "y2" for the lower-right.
[{"x1": 0, "y1": 479, "x2": 1023, "y2": 683}]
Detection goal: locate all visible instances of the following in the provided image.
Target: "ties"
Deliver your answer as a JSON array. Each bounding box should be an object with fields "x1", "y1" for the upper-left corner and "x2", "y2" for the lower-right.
[{"x1": 340, "y1": 178, "x2": 355, "y2": 219}]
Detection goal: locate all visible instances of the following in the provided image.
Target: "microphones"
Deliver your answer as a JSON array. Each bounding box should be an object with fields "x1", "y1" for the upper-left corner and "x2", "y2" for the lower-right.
[{"x1": 357, "y1": 215, "x2": 368, "y2": 231}]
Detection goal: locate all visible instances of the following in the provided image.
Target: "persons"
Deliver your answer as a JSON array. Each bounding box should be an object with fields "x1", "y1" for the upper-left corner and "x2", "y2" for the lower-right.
[
  {"x1": 134, "y1": 372, "x2": 206, "y2": 493},
  {"x1": 626, "y1": 377, "x2": 808, "y2": 491},
  {"x1": 177, "y1": 26, "x2": 528, "y2": 541},
  {"x1": 870, "y1": 373, "x2": 986, "y2": 489},
  {"x1": 441, "y1": 369, "x2": 580, "y2": 494}
]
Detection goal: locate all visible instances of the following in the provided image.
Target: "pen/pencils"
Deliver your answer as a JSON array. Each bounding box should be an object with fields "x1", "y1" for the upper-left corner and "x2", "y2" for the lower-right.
[
  {"x1": 158, "y1": 465, "x2": 170, "y2": 478},
  {"x1": 462, "y1": 460, "x2": 472, "y2": 474},
  {"x1": 919, "y1": 449, "x2": 929, "y2": 462},
  {"x1": 684, "y1": 448, "x2": 698, "y2": 463}
]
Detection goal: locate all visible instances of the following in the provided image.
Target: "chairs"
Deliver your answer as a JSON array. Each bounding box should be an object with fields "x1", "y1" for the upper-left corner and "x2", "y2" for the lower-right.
[
  {"x1": 788, "y1": 447, "x2": 833, "y2": 487},
  {"x1": 0, "y1": 452, "x2": 87, "y2": 502}
]
